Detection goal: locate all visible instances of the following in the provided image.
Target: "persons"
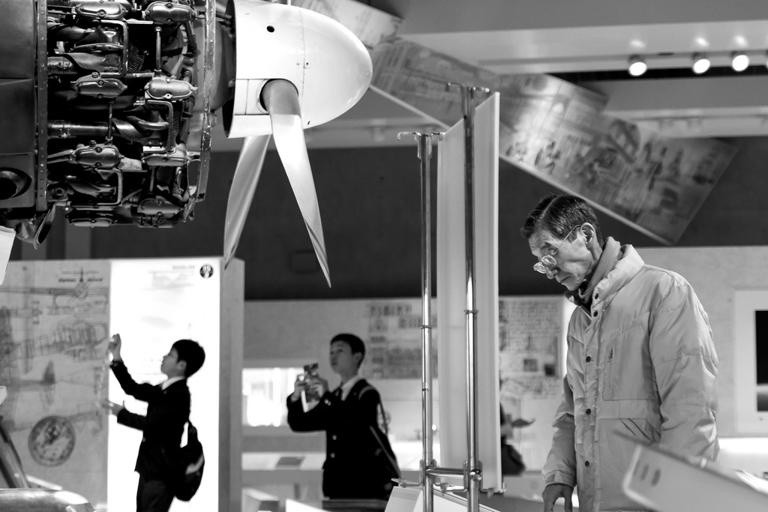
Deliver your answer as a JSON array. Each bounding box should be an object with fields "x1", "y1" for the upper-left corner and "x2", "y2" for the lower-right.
[
  {"x1": 287, "y1": 334, "x2": 402, "y2": 512},
  {"x1": 519, "y1": 193, "x2": 719, "y2": 512},
  {"x1": 500, "y1": 380, "x2": 537, "y2": 474},
  {"x1": 101, "y1": 334, "x2": 205, "y2": 512}
]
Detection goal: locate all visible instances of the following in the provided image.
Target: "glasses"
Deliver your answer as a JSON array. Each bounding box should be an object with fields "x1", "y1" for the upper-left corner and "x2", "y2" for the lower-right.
[{"x1": 533, "y1": 225, "x2": 580, "y2": 275}]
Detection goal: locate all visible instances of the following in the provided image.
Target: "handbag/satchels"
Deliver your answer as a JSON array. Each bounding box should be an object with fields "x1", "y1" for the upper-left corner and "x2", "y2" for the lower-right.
[{"x1": 162, "y1": 424, "x2": 207, "y2": 502}]
